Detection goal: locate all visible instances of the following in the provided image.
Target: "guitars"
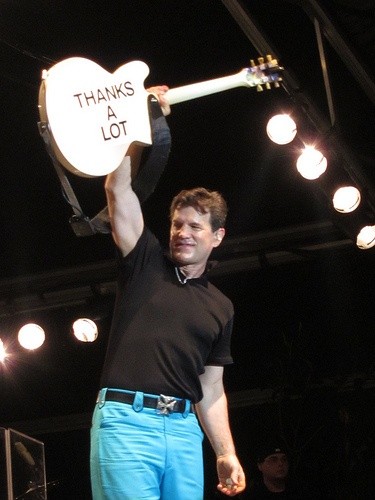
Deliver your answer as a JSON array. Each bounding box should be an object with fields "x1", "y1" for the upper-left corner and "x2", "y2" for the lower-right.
[{"x1": 39, "y1": 55, "x2": 283, "y2": 178}]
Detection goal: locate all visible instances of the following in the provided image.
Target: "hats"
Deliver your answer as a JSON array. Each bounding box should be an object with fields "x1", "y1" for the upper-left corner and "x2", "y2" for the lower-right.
[{"x1": 262, "y1": 445, "x2": 289, "y2": 462}]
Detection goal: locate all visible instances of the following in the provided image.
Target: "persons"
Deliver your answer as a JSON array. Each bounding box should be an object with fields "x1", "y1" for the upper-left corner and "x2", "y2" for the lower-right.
[
  {"x1": 86, "y1": 85, "x2": 250, "y2": 500},
  {"x1": 240, "y1": 444, "x2": 311, "y2": 500}
]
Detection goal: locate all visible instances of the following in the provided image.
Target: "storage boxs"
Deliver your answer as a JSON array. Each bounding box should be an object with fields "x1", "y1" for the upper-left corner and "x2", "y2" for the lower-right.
[{"x1": 0, "y1": 426, "x2": 48, "y2": 500}]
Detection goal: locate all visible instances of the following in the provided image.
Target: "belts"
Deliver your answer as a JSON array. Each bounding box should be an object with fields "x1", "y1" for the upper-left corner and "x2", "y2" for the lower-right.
[{"x1": 96, "y1": 390, "x2": 194, "y2": 413}]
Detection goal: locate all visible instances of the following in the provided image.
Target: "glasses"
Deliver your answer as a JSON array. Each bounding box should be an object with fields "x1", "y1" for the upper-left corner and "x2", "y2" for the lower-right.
[{"x1": 263, "y1": 456, "x2": 288, "y2": 465}]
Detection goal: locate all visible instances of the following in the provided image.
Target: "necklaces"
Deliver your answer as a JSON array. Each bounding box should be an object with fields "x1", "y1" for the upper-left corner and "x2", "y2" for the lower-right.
[{"x1": 175, "y1": 266, "x2": 198, "y2": 284}]
